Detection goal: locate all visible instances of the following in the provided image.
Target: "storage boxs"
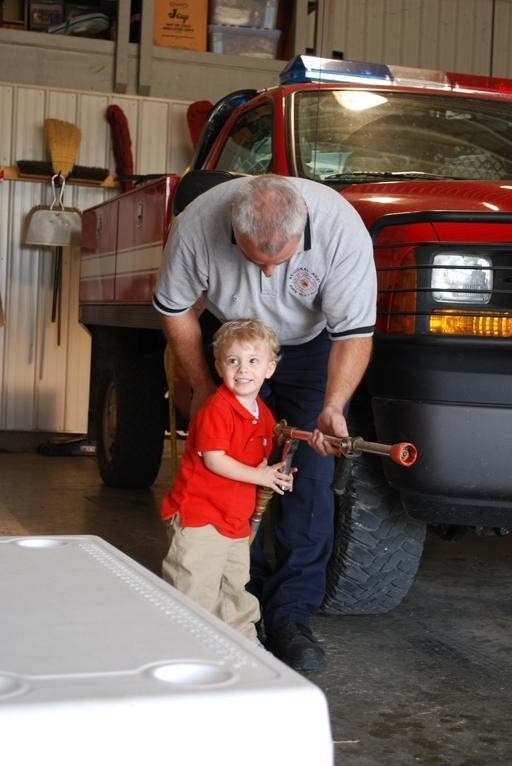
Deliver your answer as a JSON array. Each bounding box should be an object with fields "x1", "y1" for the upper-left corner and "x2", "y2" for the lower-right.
[
  {"x1": 4, "y1": 533, "x2": 333, "y2": 766},
  {"x1": 153, "y1": 0, "x2": 286, "y2": 59}
]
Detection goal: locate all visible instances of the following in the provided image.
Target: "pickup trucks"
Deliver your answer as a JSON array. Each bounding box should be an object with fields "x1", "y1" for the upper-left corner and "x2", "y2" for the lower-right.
[{"x1": 77, "y1": 55, "x2": 512, "y2": 616}]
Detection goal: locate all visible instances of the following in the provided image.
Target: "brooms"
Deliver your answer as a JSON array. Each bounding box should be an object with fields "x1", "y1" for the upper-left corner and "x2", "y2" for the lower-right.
[{"x1": 43, "y1": 118, "x2": 82, "y2": 322}]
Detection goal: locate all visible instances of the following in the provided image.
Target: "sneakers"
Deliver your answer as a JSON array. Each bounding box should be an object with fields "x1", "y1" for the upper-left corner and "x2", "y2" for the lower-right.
[{"x1": 255, "y1": 619, "x2": 328, "y2": 676}]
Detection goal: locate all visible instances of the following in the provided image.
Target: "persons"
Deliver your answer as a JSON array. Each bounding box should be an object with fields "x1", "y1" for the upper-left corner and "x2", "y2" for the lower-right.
[
  {"x1": 160, "y1": 318, "x2": 298, "y2": 644},
  {"x1": 151, "y1": 175, "x2": 379, "y2": 677}
]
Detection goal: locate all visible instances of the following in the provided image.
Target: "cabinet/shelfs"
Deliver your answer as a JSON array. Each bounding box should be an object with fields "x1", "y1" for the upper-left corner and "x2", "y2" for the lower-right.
[{"x1": 1, "y1": 0, "x2": 308, "y2": 101}]
